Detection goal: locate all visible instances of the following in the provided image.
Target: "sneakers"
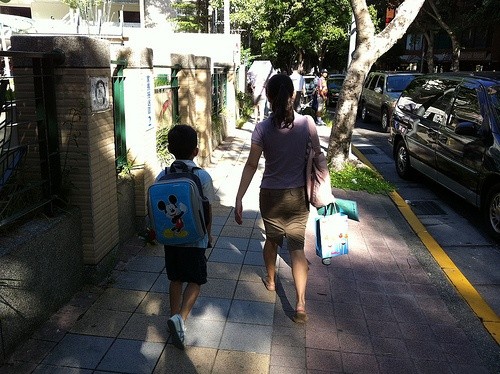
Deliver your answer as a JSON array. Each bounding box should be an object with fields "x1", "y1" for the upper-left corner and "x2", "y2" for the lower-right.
[{"x1": 167, "y1": 313, "x2": 186, "y2": 349}]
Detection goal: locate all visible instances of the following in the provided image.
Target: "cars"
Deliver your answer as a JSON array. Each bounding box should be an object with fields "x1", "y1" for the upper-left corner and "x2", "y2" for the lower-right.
[
  {"x1": 326, "y1": 74, "x2": 346, "y2": 107},
  {"x1": 301, "y1": 76, "x2": 317, "y2": 101}
]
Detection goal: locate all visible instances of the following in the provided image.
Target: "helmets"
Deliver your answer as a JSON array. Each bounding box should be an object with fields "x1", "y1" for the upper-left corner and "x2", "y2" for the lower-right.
[{"x1": 321, "y1": 69, "x2": 328, "y2": 72}]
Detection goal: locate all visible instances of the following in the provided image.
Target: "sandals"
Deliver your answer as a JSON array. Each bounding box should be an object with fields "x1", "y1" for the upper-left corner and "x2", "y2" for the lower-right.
[
  {"x1": 263, "y1": 274, "x2": 276, "y2": 291},
  {"x1": 293, "y1": 310, "x2": 307, "y2": 323}
]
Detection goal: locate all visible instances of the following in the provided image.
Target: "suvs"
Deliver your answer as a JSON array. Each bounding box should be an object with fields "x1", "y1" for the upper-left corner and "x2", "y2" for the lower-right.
[
  {"x1": 360, "y1": 72, "x2": 424, "y2": 132},
  {"x1": 388, "y1": 73, "x2": 500, "y2": 238}
]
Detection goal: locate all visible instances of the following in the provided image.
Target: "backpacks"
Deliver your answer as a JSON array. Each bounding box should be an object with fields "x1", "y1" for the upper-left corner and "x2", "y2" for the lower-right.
[{"x1": 148, "y1": 160, "x2": 209, "y2": 244}]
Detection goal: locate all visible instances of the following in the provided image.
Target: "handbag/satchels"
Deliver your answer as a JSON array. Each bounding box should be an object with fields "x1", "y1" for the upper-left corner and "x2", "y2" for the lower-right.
[
  {"x1": 316, "y1": 203, "x2": 349, "y2": 258},
  {"x1": 305, "y1": 143, "x2": 335, "y2": 209}
]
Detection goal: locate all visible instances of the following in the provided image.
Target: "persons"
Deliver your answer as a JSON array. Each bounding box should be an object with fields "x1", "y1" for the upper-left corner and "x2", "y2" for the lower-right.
[
  {"x1": 280, "y1": 64, "x2": 329, "y2": 126},
  {"x1": 155, "y1": 124, "x2": 213, "y2": 351},
  {"x1": 234, "y1": 75, "x2": 320, "y2": 325}
]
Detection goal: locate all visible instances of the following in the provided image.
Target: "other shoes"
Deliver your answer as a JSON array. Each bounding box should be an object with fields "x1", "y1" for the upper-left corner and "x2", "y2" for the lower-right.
[{"x1": 316, "y1": 121, "x2": 327, "y2": 125}]
